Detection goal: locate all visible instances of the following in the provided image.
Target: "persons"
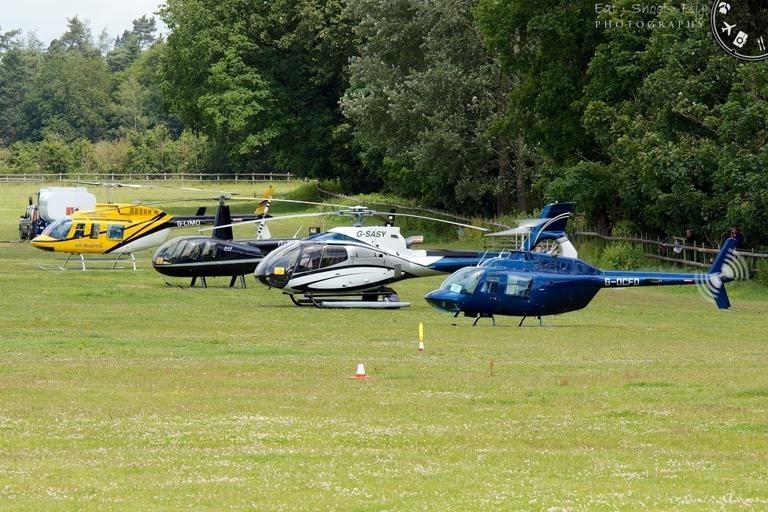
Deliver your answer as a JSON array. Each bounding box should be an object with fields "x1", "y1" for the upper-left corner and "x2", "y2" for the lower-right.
[
  {"x1": 656, "y1": 228, "x2": 699, "y2": 268},
  {"x1": 595, "y1": 218, "x2": 614, "y2": 237},
  {"x1": 726, "y1": 226, "x2": 744, "y2": 248}
]
